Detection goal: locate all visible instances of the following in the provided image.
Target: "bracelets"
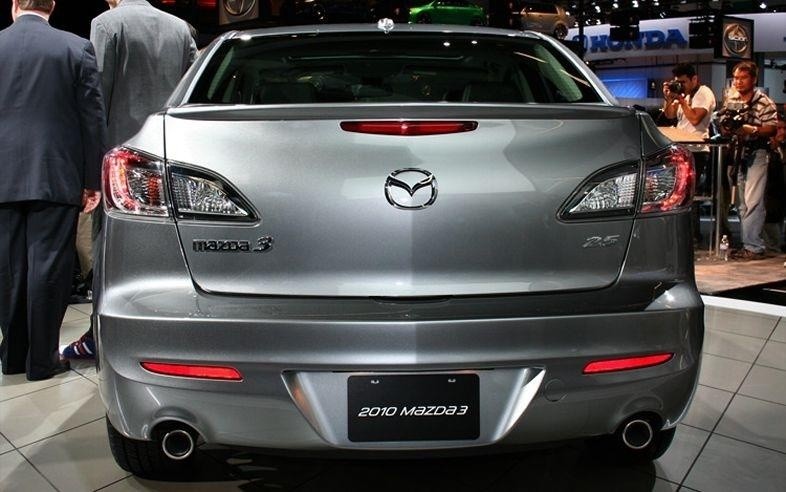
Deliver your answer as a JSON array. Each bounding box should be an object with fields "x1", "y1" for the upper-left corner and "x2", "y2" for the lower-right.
[{"x1": 752, "y1": 126, "x2": 760, "y2": 136}]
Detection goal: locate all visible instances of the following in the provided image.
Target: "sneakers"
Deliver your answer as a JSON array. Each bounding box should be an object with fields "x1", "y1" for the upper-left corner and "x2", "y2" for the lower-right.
[
  {"x1": 731, "y1": 248, "x2": 767, "y2": 262},
  {"x1": 63, "y1": 335, "x2": 96, "y2": 362}
]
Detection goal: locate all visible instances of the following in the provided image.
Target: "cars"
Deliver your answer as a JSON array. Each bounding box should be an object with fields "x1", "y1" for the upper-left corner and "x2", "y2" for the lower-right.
[
  {"x1": 92, "y1": 18, "x2": 703, "y2": 475},
  {"x1": 408, "y1": 1, "x2": 576, "y2": 38}
]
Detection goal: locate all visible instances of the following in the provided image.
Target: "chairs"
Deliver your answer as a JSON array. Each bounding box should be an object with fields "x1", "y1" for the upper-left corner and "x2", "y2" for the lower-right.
[{"x1": 250, "y1": 77, "x2": 512, "y2": 101}]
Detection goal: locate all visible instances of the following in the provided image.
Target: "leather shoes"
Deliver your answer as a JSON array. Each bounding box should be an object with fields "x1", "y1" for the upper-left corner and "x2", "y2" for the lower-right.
[{"x1": 26, "y1": 359, "x2": 70, "y2": 381}]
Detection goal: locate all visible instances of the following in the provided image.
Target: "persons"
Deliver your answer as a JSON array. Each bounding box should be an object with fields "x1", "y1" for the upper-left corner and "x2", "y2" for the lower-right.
[
  {"x1": 712, "y1": 61, "x2": 779, "y2": 262},
  {"x1": 0, "y1": 0, "x2": 104, "y2": 384},
  {"x1": 662, "y1": 60, "x2": 718, "y2": 250},
  {"x1": 60, "y1": 0, "x2": 198, "y2": 361},
  {"x1": 763, "y1": 117, "x2": 786, "y2": 256}
]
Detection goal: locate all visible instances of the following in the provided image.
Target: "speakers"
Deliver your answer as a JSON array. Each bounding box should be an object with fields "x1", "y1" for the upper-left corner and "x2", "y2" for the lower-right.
[
  {"x1": 610, "y1": 27, "x2": 640, "y2": 41},
  {"x1": 689, "y1": 35, "x2": 714, "y2": 49},
  {"x1": 688, "y1": 22, "x2": 715, "y2": 34},
  {"x1": 610, "y1": 11, "x2": 639, "y2": 26}
]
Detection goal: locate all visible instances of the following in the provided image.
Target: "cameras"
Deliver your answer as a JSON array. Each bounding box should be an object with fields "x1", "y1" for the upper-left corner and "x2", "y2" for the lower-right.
[{"x1": 668, "y1": 80, "x2": 682, "y2": 93}]
